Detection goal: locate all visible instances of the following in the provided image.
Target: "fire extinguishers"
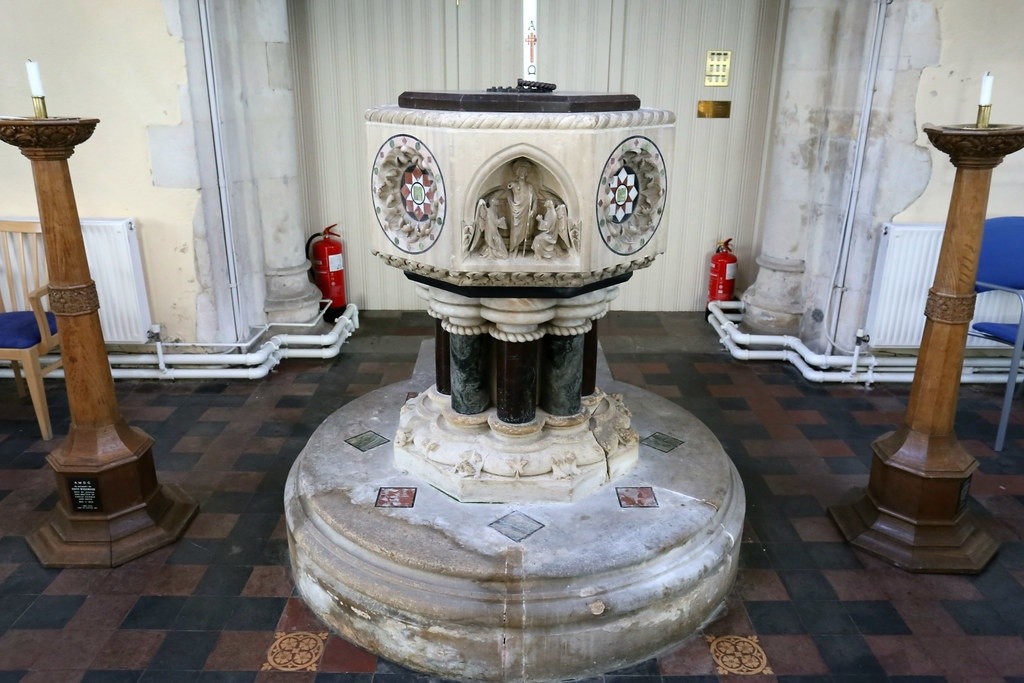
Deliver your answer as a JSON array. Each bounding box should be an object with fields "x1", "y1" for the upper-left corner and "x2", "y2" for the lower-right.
[
  {"x1": 305, "y1": 223, "x2": 347, "y2": 323},
  {"x1": 705, "y1": 238, "x2": 738, "y2": 322}
]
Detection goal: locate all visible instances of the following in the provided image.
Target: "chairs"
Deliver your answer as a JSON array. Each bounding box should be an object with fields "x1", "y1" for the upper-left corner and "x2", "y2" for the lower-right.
[
  {"x1": 965, "y1": 217, "x2": 1024, "y2": 455},
  {"x1": 0, "y1": 222, "x2": 62, "y2": 441}
]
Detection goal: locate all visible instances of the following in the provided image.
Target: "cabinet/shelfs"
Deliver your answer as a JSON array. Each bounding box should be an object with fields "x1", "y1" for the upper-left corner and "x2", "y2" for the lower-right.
[{"x1": 862, "y1": 219, "x2": 1024, "y2": 348}]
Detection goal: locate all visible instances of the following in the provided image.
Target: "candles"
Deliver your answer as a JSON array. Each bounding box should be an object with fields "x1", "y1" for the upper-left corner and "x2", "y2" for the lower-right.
[
  {"x1": 24, "y1": 56, "x2": 45, "y2": 97},
  {"x1": 978, "y1": 70, "x2": 994, "y2": 105},
  {"x1": 523, "y1": 0, "x2": 538, "y2": 90}
]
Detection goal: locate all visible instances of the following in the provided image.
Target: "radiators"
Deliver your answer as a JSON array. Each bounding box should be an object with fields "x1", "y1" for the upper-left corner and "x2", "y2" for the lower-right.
[{"x1": 0, "y1": 216, "x2": 154, "y2": 346}]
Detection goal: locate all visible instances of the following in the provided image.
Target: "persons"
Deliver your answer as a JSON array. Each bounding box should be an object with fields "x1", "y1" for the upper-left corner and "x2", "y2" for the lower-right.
[{"x1": 507, "y1": 161, "x2": 538, "y2": 254}]
